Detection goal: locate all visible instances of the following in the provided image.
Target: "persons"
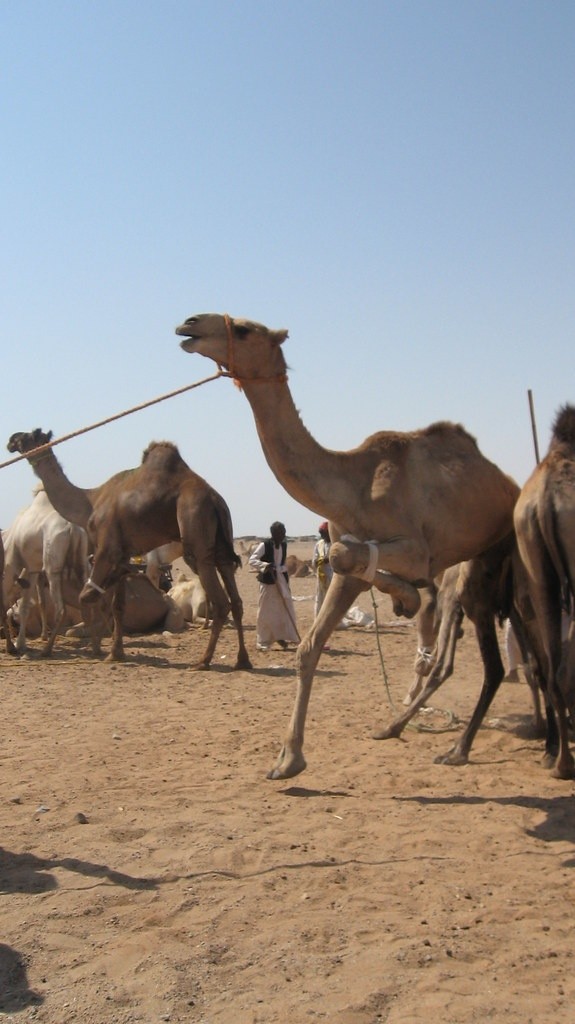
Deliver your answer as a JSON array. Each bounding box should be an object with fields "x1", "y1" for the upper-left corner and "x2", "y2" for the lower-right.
[
  {"x1": 249, "y1": 521, "x2": 298, "y2": 651},
  {"x1": 312, "y1": 522, "x2": 333, "y2": 621},
  {"x1": 499, "y1": 616, "x2": 525, "y2": 683}
]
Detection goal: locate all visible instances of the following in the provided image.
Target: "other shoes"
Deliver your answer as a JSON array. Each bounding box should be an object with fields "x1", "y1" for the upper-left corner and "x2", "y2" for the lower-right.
[
  {"x1": 256, "y1": 643, "x2": 269, "y2": 651},
  {"x1": 277, "y1": 640, "x2": 288, "y2": 648},
  {"x1": 323, "y1": 644, "x2": 330, "y2": 650}
]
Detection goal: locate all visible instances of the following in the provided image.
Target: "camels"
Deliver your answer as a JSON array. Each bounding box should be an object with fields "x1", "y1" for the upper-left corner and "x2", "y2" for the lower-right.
[
  {"x1": 0, "y1": 429, "x2": 251, "y2": 673},
  {"x1": 176, "y1": 313, "x2": 575, "y2": 780}
]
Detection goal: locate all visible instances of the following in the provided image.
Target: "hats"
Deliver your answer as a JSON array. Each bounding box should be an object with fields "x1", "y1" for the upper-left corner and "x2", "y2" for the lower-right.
[{"x1": 320, "y1": 521, "x2": 328, "y2": 532}]
